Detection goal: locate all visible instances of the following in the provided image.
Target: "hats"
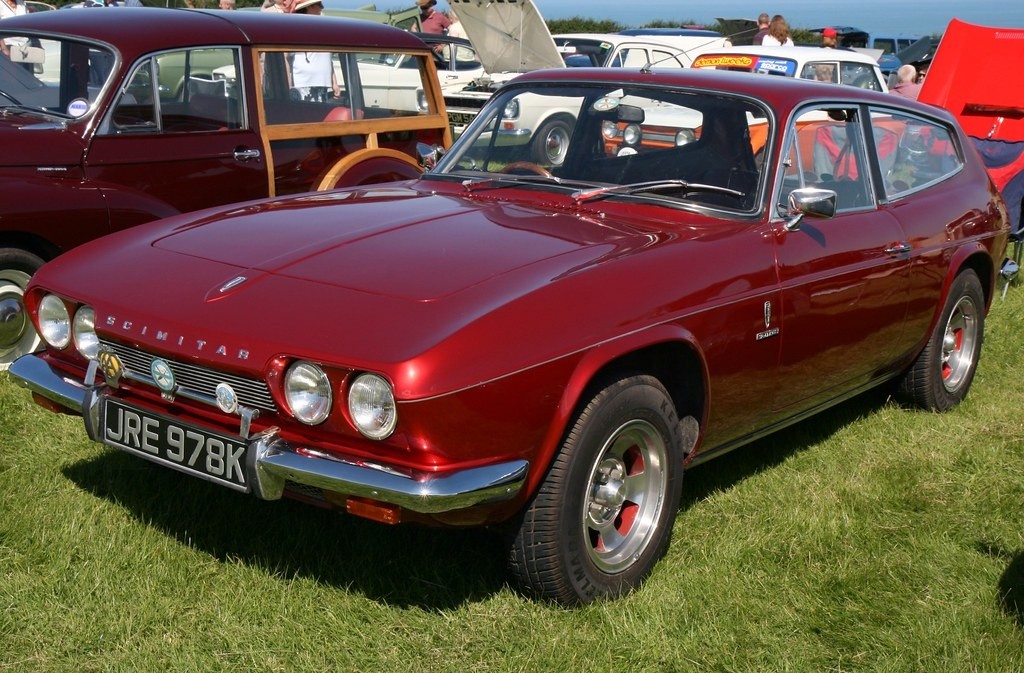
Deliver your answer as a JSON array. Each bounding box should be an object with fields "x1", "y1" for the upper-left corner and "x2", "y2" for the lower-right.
[
  {"x1": 294, "y1": 0, "x2": 321, "y2": 10},
  {"x1": 821, "y1": 28, "x2": 837, "y2": 38}
]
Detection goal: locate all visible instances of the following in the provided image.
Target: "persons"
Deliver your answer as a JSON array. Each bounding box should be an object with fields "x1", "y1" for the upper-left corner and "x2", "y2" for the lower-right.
[
  {"x1": 753, "y1": 14, "x2": 794, "y2": 47},
  {"x1": 84, "y1": 0, "x2": 144, "y2": 87},
  {"x1": 261, "y1": 0, "x2": 341, "y2": 103},
  {"x1": 814, "y1": 27, "x2": 838, "y2": 82},
  {"x1": 0, "y1": 0, "x2": 34, "y2": 75},
  {"x1": 410, "y1": 0, "x2": 475, "y2": 62},
  {"x1": 219, "y1": 0, "x2": 235, "y2": 10},
  {"x1": 890, "y1": 64, "x2": 930, "y2": 101}
]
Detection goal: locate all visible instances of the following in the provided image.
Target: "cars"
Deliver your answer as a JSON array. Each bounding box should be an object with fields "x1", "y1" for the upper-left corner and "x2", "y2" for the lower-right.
[
  {"x1": 7, "y1": 67, "x2": 1008, "y2": 613},
  {"x1": 214, "y1": 17, "x2": 1024, "y2": 266},
  {"x1": 1, "y1": 7, "x2": 452, "y2": 373}
]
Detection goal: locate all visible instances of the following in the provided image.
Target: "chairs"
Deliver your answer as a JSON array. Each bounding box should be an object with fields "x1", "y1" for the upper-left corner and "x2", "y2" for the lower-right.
[
  {"x1": 172, "y1": 93, "x2": 236, "y2": 131},
  {"x1": 813, "y1": 125, "x2": 899, "y2": 184},
  {"x1": 943, "y1": 134, "x2": 1024, "y2": 301}
]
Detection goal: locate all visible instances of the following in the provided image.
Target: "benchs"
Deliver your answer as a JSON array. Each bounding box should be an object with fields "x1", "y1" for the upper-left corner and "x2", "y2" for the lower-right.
[{"x1": 265, "y1": 99, "x2": 364, "y2": 123}]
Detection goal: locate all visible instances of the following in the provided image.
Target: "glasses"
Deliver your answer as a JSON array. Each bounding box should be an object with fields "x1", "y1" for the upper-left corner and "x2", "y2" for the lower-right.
[
  {"x1": 420, "y1": 3, "x2": 430, "y2": 10},
  {"x1": 919, "y1": 74, "x2": 926, "y2": 77}
]
